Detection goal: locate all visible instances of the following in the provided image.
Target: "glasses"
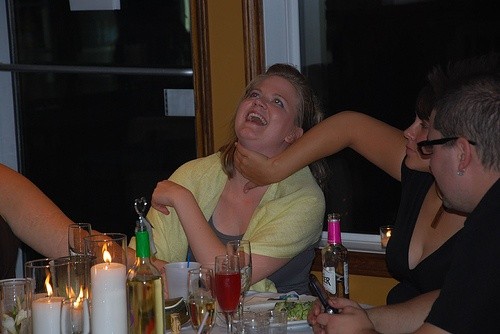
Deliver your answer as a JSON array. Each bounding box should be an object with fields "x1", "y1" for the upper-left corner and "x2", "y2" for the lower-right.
[{"x1": 417, "y1": 137, "x2": 478, "y2": 159}]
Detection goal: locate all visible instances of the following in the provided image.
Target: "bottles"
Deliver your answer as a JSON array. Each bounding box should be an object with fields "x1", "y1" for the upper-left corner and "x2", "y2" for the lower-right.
[
  {"x1": 126, "y1": 230, "x2": 167, "y2": 334},
  {"x1": 322, "y1": 213, "x2": 349, "y2": 313}
]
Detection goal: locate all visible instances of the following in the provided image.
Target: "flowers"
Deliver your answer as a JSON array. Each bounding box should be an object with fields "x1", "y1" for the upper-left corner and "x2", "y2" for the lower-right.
[{"x1": 1, "y1": 296, "x2": 31, "y2": 333}]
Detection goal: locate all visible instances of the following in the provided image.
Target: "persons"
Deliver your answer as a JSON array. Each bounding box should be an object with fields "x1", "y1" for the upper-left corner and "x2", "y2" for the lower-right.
[
  {"x1": 230, "y1": 79, "x2": 500, "y2": 334},
  {"x1": 127, "y1": 62, "x2": 326, "y2": 300},
  {"x1": 0, "y1": 163, "x2": 214, "y2": 297}
]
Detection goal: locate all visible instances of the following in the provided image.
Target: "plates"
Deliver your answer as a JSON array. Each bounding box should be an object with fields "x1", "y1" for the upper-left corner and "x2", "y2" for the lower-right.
[{"x1": 243, "y1": 300, "x2": 308, "y2": 327}]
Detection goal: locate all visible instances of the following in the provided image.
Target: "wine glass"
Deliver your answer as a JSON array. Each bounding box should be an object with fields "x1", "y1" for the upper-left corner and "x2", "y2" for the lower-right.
[{"x1": 187, "y1": 240, "x2": 252, "y2": 334}]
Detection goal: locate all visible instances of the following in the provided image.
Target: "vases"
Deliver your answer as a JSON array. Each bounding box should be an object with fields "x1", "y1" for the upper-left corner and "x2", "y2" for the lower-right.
[{"x1": 0, "y1": 278, "x2": 34, "y2": 333}]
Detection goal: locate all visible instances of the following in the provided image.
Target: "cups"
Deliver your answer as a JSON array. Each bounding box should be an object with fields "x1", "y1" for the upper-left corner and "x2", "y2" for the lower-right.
[
  {"x1": 379, "y1": 225, "x2": 392, "y2": 249},
  {"x1": 0, "y1": 223, "x2": 129, "y2": 334},
  {"x1": 238, "y1": 309, "x2": 288, "y2": 334},
  {"x1": 164, "y1": 262, "x2": 202, "y2": 298}
]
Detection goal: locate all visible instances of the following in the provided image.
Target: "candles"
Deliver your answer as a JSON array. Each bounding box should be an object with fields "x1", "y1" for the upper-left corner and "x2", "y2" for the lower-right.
[
  {"x1": 31, "y1": 233, "x2": 133, "y2": 333},
  {"x1": 379, "y1": 225, "x2": 395, "y2": 249}
]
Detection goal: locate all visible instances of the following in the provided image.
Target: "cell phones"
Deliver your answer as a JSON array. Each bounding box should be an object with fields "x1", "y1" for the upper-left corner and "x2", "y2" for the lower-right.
[{"x1": 309, "y1": 274, "x2": 339, "y2": 314}]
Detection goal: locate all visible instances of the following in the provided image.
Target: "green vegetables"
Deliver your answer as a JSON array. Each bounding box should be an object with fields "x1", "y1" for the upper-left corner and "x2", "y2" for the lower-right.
[{"x1": 272, "y1": 300, "x2": 313, "y2": 321}]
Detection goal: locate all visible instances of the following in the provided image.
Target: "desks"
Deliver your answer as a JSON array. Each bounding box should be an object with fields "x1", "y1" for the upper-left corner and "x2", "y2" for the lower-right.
[{"x1": 0, "y1": 291, "x2": 372, "y2": 333}]
